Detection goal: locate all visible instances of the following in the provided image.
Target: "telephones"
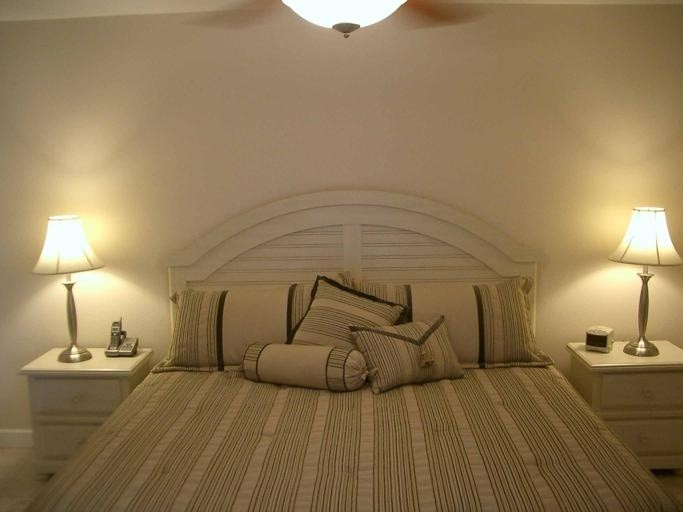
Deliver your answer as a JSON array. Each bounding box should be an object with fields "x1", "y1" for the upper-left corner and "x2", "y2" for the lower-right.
[{"x1": 105, "y1": 316, "x2": 138, "y2": 357}]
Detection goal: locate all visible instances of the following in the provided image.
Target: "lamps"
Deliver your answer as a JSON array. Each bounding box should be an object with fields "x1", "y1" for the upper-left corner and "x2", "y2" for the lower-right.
[
  {"x1": 607, "y1": 205, "x2": 683, "y2": 358},
  {"x1": 30, "y1": 214, "x2": 104, "y2": 363},
  {"x1": 279, "y1": 0, "x2": 409, "y2": 40}
]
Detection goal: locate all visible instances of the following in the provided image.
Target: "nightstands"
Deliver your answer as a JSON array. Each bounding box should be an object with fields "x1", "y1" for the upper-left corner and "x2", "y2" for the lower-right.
[
  {"x1": 565, "y1": 340, "x2": 683, "y2": 469},
  {"x1": 15, "y1": 346, "x2": 154, "y2": 481}
]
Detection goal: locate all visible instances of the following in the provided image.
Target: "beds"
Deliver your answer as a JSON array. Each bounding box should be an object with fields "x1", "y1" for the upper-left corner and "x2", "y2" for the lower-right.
[{"x1": 22, "y1": 189, "x2": 683, "y2": 512}]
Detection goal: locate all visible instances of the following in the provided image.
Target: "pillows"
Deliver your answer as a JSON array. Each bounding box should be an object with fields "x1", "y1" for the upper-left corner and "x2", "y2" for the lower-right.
[
  {"x1": 150, "y1": 272, "x2": 352, "y2": 373},
  {"x1": 353, "y1": 276, "x2": 554, "y2": 369},
  {"x1": 348, "y1": 315, "x2": 465, "y2": 393},
  {"x1": 238, "y1": 342, "x2": 367, "y2": 393},
  {"x1": 285, "y1": 276, "x2": 407, "y2": 348}
]
